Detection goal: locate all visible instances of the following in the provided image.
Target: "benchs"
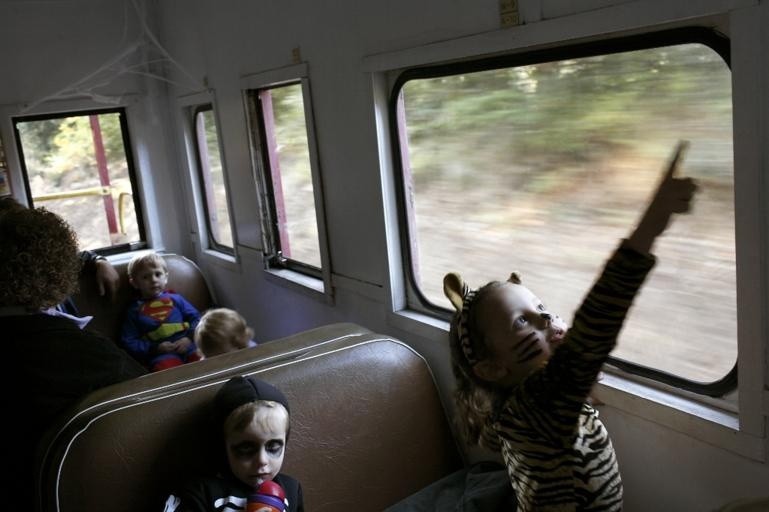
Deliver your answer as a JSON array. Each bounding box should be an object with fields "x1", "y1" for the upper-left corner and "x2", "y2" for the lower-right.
[
  {"x1": 40, "y1": 331, "x2": 463, "y2": 509},
  {"x1": 36, "y1": 314, "x2": 377, "y2": 512},
  {"x1": 60, "y1": 245, "x2": 220, "y2": 348}
]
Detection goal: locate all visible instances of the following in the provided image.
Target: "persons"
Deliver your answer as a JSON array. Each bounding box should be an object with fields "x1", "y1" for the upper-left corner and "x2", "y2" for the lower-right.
[
  {"x1": 0, "y1": 206, "x2": 150, "y2": 512},
  {"x1": 193, "y1": 307, "x2": 259, "y2": 361},
  {"x1": 119, "y1": 252, "x2": 203, "y2": 370},
  {"x1": 442, "y1": 140, "x2": 696, "y2": 511},
  {"x1": 1, "y1": 196, "x2": 122, "y2": 318},
  {"x1": 157, "y1": 375, "x2": 304, "y2": 512}
]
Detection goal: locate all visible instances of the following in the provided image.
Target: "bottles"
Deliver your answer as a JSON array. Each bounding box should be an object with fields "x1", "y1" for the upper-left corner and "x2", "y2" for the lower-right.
[{"x1": 246, "y1": 480, "x2": 285, "y2": 512}]
